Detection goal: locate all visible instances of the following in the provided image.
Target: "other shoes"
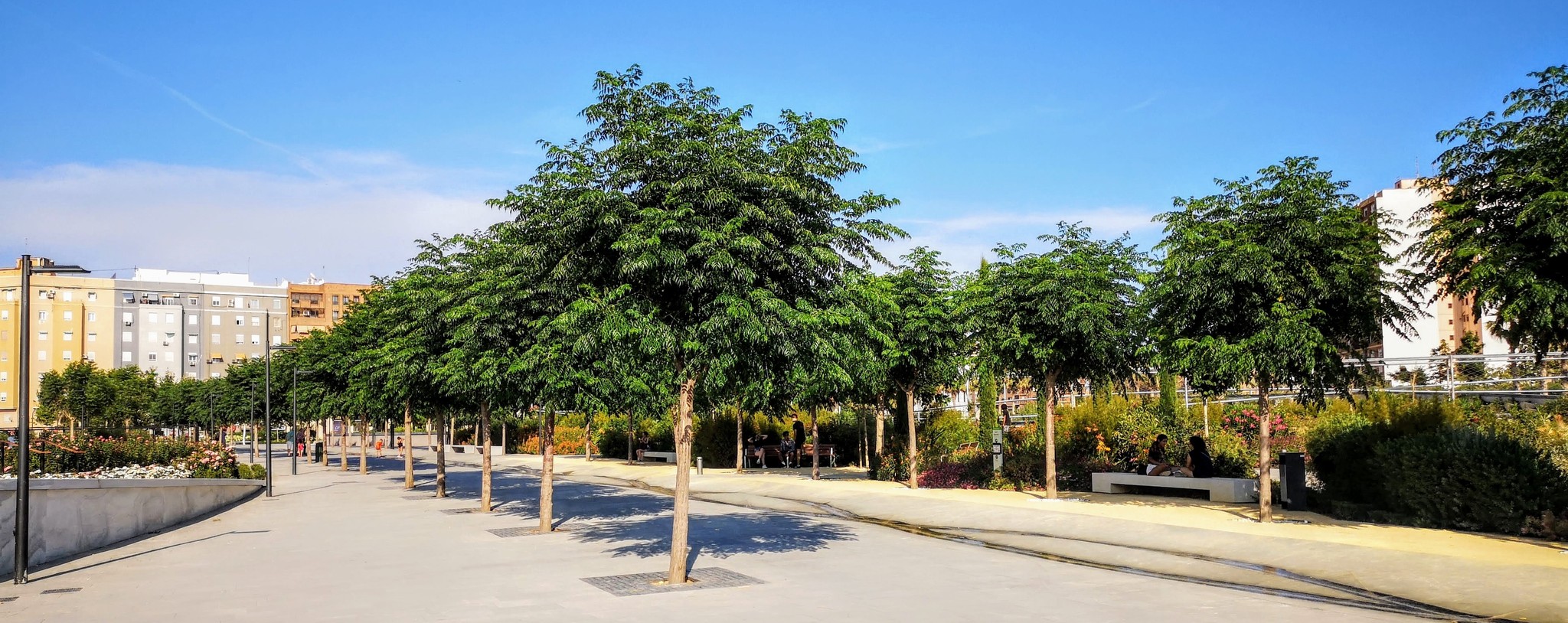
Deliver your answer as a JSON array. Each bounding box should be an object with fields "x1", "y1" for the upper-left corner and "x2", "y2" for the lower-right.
[
  {"x1": 801, "y1": 453, "x2": 806, "y2": 457},
  {"x1": 380, "y1": 456, "x2": 382, "y2": 457},
  {"x1": 793, "y1": 465, "x2": 800, "y2": 468},
  {"x1": 1159, "y1": 471, "x2": 1173, "y2": 476},
  {"x1": 637, "y1": 459, "x2": 641, "y2": 462},
  {"x1": 1175, "y1": 471, "x2": 1188, "y2": 477},
  {"x1": 376, "y1": 457, "x2": 378, "y2": 458},
  {"x1": 397, "y1": 456, "x2": 403, "y2": 458}
]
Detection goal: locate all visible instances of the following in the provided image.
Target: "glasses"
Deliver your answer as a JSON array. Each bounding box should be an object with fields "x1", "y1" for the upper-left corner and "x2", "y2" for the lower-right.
[
  {"x1": 757, "y1": 435, "x2": 759, "y2": 439},
  {"x1": 784, "y1": 434, "x2": 789, "y2": 437}
]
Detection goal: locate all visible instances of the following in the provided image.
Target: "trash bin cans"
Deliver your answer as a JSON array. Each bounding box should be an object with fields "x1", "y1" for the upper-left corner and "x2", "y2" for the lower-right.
[
  {"x1": 697, "y1": 457, "x2": 703, "y2": 475},
  {"x1": 315, "y1": 442, "x2": 323, "y2": 463},
  {"x1": 1278, "y1": 452, "x2": 1305, "y2": 511}
]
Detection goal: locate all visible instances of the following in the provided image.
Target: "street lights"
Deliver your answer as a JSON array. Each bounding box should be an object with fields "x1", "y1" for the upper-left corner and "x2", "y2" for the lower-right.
[
  {"x1": 250, "y1": 381, "x2": 261, "y2": 463},
  {"x1": 292, "y1": 370, "x2": 315, "y2": 476},
  {"x1": 266, "y1": 347, "x2": 296, "y2": 497},
  {"x1": 211, "y1": 394, "x2": 222, "y2": 440},
  {"x1": 14, "y1": 264, "x2": 91, "y2": 583}
]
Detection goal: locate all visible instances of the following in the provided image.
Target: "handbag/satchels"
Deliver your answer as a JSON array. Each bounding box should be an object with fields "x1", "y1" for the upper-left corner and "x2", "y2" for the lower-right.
[
  {"x1": 1137, "y1": 463, "x2": 1148, "y2": 475},
  {"x1": 297, "y1": 440, "x2": 300, "y2": 443},
  {"x1": 640, "y1": 441, "x2": 647, "y2": 450}
]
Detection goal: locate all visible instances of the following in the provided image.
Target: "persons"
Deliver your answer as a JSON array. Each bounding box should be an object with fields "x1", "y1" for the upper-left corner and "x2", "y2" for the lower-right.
[
  {"x1": 791, "y1": 413, "x2": 806, "y2": 468},
  {"x1": 1001, "y1": 404, "x2": 1011, "y2": 432},
  {"x1": 778, "y1": 431, "x2": 795, "y2": 469},
  {"x1": 1146, "y1": 434, "x2": 1215, "y2": 478},
  {"x1": 285, "y1": 428, "x2": 307, "y2": 457},
  {"x1": 397, "y1": 437, "x2": 405, "y2": 458},
  {"x1": 889, "y1": 408, "x2": 898, "y2": 448},
  {"x1": 747, "y1": 433, "x2": 768, "y2": 469},
  {"x1": 219, "y1": 430, "x2": 226, "y2": 448},
  {"x1": 376, "y1": 439, "x2": 383, "y2": 458},
  {"x1": 636, "y1": 431, "x2": 651, "y2": 462}
]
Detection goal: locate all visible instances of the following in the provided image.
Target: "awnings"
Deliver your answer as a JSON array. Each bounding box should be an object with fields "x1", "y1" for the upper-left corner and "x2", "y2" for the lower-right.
[
  {"x1": 211, "y1": 353, "x2": 222, "y2": 358},
  {"x1": 235, "y1": 353, "x2": 246, "y2": 360},
  {"x1": 291, "y1": 325, "x2": 325, "y2": 334}
]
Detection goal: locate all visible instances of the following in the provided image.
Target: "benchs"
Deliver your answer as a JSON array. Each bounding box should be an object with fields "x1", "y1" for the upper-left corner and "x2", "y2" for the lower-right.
[
  {"x1": 1089, "y1": 471, "x2": 1260, "y2": 502},
  {"x1": 449, "y1": 445, "x2": 475, "y2": 454},
  {"x1": 429, "y1": 445, "x2": 451, "y2": 453},
  {"x1": 474, "y1": 445, "x2": 502, "y2": 455},
  {"x1": 744, "y1": 445, "x2": 788, "y2": 468},
  {"x1": 787, "y1": 444, "x2": 836, "y2": 467},
  {"x1": 957, "y1": 440, "x2": 980, "y2": 453},
  {"x1": 637, "y1": 450, "x2": 676, "y2": 464}
]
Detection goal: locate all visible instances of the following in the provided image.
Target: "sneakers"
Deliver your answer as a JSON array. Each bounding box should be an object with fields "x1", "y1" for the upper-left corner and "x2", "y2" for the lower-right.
[
  {"x1": 779, "y1": 464, "x2": 786, "y2": 468},
  {"x1": 788, "y1": 464, "x2": 793, "y2": 468},
  {"x1": 757, "y1": 459, "x2": 760, "y2": 464},
  {"x1": 762, "y1": 465, "x2": 768, "y2": 469}
]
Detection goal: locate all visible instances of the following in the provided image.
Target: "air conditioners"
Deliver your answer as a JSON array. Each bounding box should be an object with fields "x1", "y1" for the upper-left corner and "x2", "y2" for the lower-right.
[
  {"x1": 228, "y1": 303, "x2": 235, "y2": 307},
  {"x1": 47, "y1": 294, "x2": 54, "y2": 298},
  {"x1": 82, "y1": 354, "x2": 88, "y2": 359},
  {"x1": 163, "y1": 341, "x2": 169, "y2": 345},
  {"x1": 125, "y1": 322, "x2": 130, "y2": 325},
  {"x1": 190, "y1": 361, "x2": 195, "y2": 365},
  {"x1": 303, "y1": 310, "x2": 309, "y2": 316},
  {"x1": 228, "y1": 300, "x2": 234, "y2": 303},
  {"x1": 207, "y1": 359, "x2": 212, "y2": 363},
  {"x1": 173, "y1": 292, "x2": 180, "y2": 297},
  {"x1": 49, "y1": 290, "x2": 55, "y2": 294},
  {"x1": 141, "y1": 292, "x2": 148, "y2": 297},
  {"x1": 307, "y1": 278, "x2": 314, "y2": 285},
  {"x1": 232, "y1": 360, "x2": 238, "y2": 363}
]
